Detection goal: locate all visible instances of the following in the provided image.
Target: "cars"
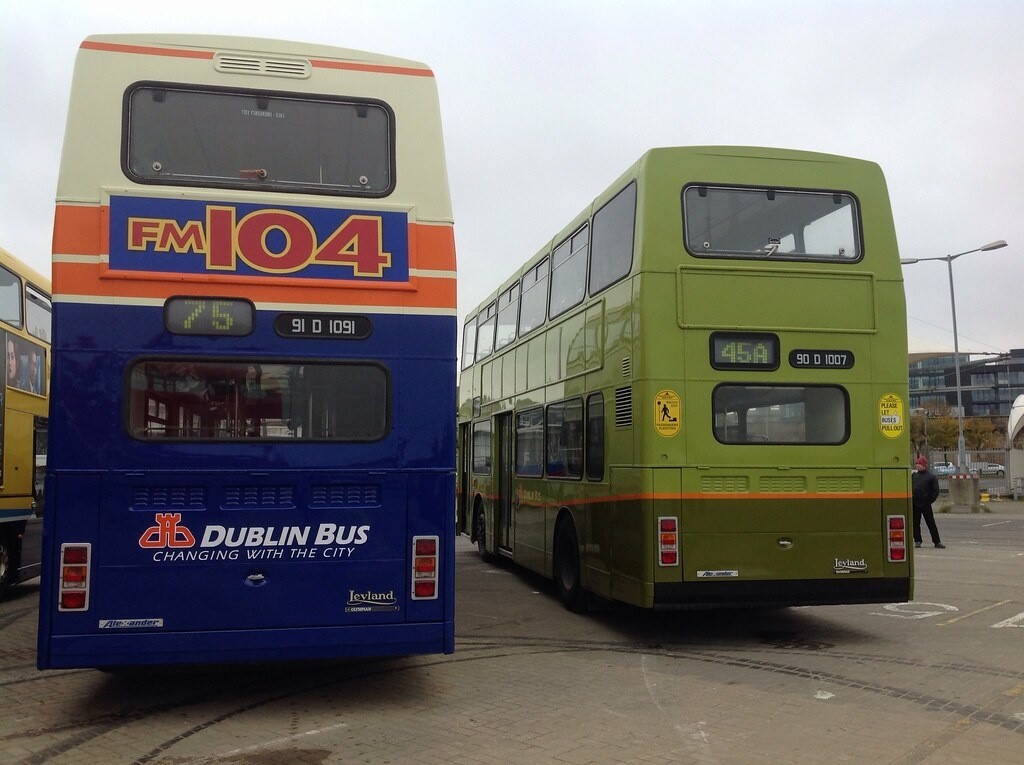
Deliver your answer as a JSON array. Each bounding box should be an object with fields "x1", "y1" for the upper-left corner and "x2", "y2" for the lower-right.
[{"x1": 968, "y1": 463, "x2": 1004, "y2": 476}]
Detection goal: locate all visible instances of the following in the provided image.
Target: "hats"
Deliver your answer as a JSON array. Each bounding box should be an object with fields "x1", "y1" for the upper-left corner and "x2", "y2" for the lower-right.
[{"x1": 916, "y1": 456, "x2": 928, "y2": 468}]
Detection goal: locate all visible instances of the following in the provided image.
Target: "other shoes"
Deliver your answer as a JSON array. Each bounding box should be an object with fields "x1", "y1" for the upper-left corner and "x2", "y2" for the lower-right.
[
  {"x1": 934, "y1": 542, "x2": 946, "y2": 549},
  {"x1": 915, "y1": 542, "x2": 921, "y2": 548}
]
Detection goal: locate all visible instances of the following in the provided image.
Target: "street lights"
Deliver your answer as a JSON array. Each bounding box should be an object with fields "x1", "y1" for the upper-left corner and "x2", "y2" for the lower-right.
[{"x1": 902, "y1": 240, "x2": 1010, "y2": 508}]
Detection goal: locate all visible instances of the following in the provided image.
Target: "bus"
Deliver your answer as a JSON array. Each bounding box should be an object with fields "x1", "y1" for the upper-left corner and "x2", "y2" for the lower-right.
[
  {"x1": 35, "y1": 32, "x2": 460, "y2": 676},
  {"x1": 0, "y1": 247, "x2": 52, "y2": 591},
  {"x1": 457, "y1": 145, "x2": 916, "y2": 616}
]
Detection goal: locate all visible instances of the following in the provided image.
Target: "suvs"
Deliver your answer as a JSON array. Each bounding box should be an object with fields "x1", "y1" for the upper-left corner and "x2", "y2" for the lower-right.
[{"x1": 911, "y1": 462, "x2": 956, "y2": 476}]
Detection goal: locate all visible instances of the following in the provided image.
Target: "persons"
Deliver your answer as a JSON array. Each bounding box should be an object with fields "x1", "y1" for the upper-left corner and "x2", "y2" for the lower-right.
[
  {"x1": 7, "y1": 336, "x2": 38, "y2": 394},
  {"x1": 911, "y1": 457, "x2": 945, "y2": 549}
]
